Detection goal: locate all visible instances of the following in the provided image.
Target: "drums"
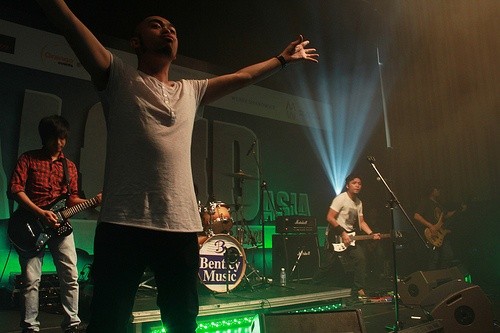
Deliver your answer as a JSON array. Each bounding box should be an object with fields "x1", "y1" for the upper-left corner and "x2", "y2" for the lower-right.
[
  {"x1": 200, "y1": 202, "x2": 234, "y2": 235},
  {"x1": 195, "y1": 233, "x2": 247, "y2": 293}
]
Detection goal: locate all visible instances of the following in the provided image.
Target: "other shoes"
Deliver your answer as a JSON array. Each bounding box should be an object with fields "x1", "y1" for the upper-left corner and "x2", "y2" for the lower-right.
[
  {"x1": 357, "y1": 289, "x2": 367, "y2": 298},
  {"x1": 61, "y1": 323, "x2": 88, "y2": 333}
]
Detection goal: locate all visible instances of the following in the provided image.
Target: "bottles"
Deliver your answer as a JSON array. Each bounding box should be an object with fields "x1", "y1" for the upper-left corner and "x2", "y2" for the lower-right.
[{"x1": 280, "y1": 267, "x2": 286, "y2": 286}]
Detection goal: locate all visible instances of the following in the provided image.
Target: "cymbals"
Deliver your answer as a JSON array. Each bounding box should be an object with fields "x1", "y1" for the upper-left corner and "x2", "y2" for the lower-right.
[
  {"x1": 227, "y1": 200, "x2": 251, "y2": 208},
  {"x1": 227, "y1": 172, "x2": 259, "y2": 181}
]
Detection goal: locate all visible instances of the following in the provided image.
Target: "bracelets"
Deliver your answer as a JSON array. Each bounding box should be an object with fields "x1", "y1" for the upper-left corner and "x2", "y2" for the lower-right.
[
  {"x1": 336, "y1": 225, "x2": 345, "y2": 234},
  {"x1": 370, "y1": 233, "x2": 373, "y2": 235},
  {"x1": 275, "y1": 55, "x2": 287, "y2": 67}
]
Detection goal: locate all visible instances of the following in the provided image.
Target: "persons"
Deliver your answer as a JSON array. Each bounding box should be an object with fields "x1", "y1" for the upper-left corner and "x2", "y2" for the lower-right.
[
  {"x1": 415, "y1": 184, "x2": 472, "y2": 274},
  {"x1": 10, "y1": 114, "x2": 103, "y2": 333},
  {"x1": 40, "y1": 0, "x2": 320, "y2": 333},
  {"x1": 194, "y1": 184, "x2": 208, "y2": 243},
  {"x1": 325, "y1": 174, "x2": 382, "y2": 297}
]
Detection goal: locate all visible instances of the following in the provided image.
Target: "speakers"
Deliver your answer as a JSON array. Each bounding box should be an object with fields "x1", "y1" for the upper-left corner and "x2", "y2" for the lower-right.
[
  {"x1": 417, "y1": 281, "x2": 492, "y2": 333},
  {"x1": 396, "y1": 266, "x2": 462, "y2": 304},
  {"x1": 250, "y1": 306, "x2": 366, "y2": 333},
  {"x1": 272, "y1": 234, "x2": 320, "y2": 282}
]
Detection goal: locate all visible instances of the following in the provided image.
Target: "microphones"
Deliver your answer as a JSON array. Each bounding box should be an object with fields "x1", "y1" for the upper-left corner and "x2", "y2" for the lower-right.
[{"x1": 246, "y1": 139, "x2": 256, "y2": 156}]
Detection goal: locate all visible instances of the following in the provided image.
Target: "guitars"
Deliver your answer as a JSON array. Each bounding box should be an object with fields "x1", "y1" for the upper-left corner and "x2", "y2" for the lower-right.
[
  {"x1": 7, "y1": 195, "x2": 99, "y2": 258},
  {"x1": 424, "y1": 204, "x2": 467, "y2": 249},
  {"x1": 328, "y1": 227, "x2": 403, "y2": 254}
]
